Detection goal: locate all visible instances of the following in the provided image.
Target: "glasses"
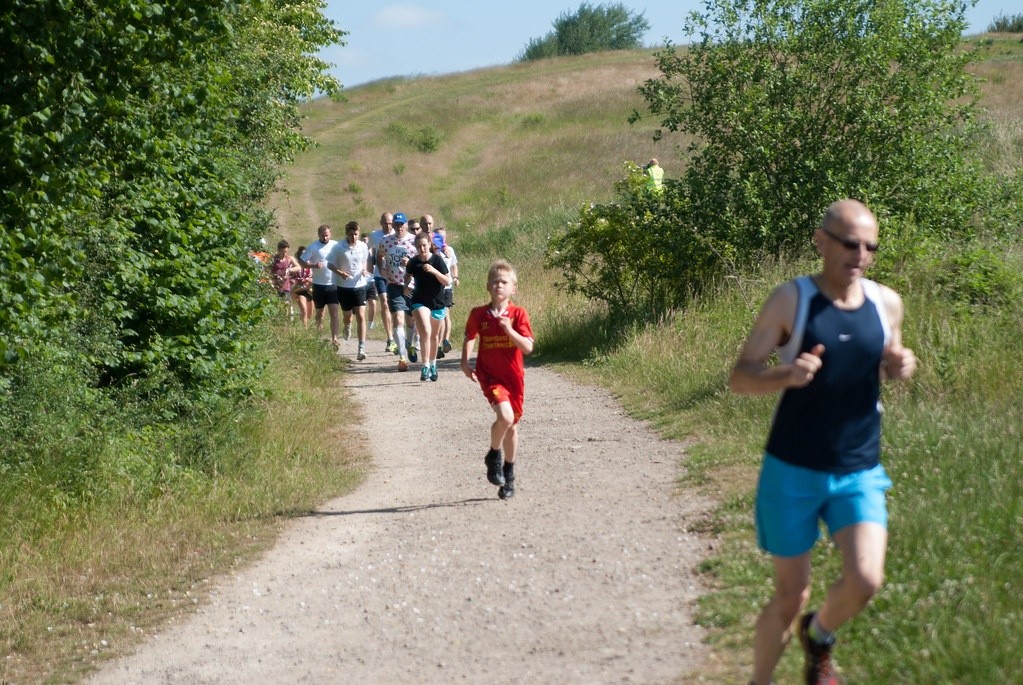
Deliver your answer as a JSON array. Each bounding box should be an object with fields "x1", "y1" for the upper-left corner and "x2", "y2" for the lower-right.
[
  {"x1": 411, "y1": 228, "x2": 419, "y2": 231},
  {"x1": 823, "y1": 230, "x2": 879, "y2": 251}
]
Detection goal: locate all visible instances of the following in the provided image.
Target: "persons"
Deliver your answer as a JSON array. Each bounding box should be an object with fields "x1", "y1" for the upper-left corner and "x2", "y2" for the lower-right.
[
  {"x1": 729, "y1": 200, "x2": 915, "y2": 685},
  {"x1": 461, "y1": 260, "x2": 534, "y2": 501},
  {"x1": 248, "y1": 212, "x2": 460, "y2": 381},
  {"x1": 643, "y1": 158, "x2": 664, "y2": 190}
]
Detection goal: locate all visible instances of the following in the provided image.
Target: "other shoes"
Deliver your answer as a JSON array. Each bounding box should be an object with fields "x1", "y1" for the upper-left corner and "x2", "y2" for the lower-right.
[
  {"x1": 368, "y1": 321, "x2": 373, "y2": 329},
  {"x1": 398, "y1": 358, "x2": 408, "y2": 372},
  {"x1": 799, "y1": 612, "x2": 835, "y2": 685},
  {"x1": 444, "y1": 338, "x2": 452, "y2": 353},
  {"x1": 437, "y1": 347, "x2": 444, "y2": 359},
  {"x1": 385, "y1": 338, "x2": 397, "y2": 351},
  {"x1": 421, "y1": 365, "x2": 438, "y2": 382},
  {"x1": 343, "y1": 324, "x2": 351, "y2": 340},
  {"x1": 357, "y1": 349, "x2": 367, "y2": 360},
  {"x1": 405, "y1": 339, "x2": 417, "y2": 363}
]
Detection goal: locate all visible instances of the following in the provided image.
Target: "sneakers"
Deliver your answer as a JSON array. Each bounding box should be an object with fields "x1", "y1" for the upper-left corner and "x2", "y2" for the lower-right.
[{"x1": 485, "y1": 452, "x2": 515, "y2": 499}]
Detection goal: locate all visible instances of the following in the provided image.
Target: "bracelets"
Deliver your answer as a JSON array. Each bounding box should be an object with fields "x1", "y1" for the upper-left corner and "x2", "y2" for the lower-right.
[{"x1": 453, "y1": 275, "x2": 458, "y2": 280}]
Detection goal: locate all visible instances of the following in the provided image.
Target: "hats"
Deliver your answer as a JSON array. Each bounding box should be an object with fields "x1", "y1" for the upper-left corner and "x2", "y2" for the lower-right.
[{"x1": 393, "y1": 213, "x2": 406, "y2": 224}]
